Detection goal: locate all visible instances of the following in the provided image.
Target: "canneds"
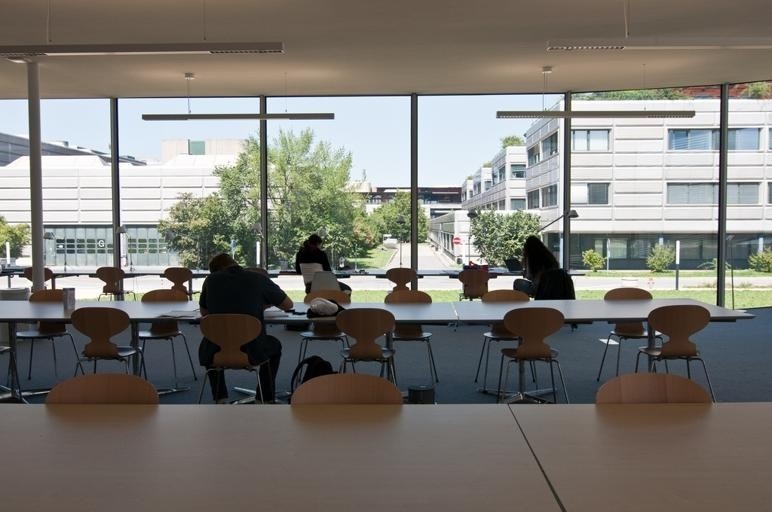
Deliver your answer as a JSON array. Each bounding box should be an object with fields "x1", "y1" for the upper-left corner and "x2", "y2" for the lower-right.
[{"x1": 62, "y1": 287, "x2": 75, "y2": 311}]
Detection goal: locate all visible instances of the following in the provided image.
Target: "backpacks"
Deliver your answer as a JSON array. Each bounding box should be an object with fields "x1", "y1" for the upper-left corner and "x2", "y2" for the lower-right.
[{"x1": 290, "y1": 355, "x2": 332, "y2": 400}]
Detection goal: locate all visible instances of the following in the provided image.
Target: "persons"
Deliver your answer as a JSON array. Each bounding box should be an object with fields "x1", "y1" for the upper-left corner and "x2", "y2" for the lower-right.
[
  {"x1": 296, "y1": 233, "x2": 352, "y2": 297},
  {"x1": 513, "y1": 236, "x2": 559, "y2": 299},
  {"x1": 199, "y1": 253, "x2": 293, "y2": 404}
]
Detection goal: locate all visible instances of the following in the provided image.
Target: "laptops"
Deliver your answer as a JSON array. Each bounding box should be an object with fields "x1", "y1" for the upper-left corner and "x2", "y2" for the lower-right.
[{"x1": 503, "y1": 258, "x2": 525, "y2": 273}]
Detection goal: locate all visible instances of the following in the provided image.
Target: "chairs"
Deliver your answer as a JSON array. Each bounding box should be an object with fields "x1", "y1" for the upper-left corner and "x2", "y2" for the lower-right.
[
  {"x1": 128, "y1": 289, "x2": 198, "y2": 386},
  {"x1": 300, "y1": 263, "x2": 323, "y2": 287},
  {"x1": 535, "y1": 269, "x2": 574, "y2": 302},
  {"x1": 596, "y1": 373, "x2": 712, "y2": 403},
  {"x1": 165, "y1": 267, "x2": 193, "y2": 293},
  {"x1": 15, "y1": 289, "x2": 85, "y2": 378},
  {"x1": 475, "y1": 289, "x2": 537, "y2": 386},
  {"x1": 44, "y1": 374, "x2": 159, "y2": 403},
  {"x1": 337, "y1": 308, "x2": 397, "y2": 384},
  {"x1": 458, "y1": 270, "x2": 490, "y2": 301},
  {"x1": 635, "y1": 304, "x2": 718, "y2": 403},
  {"x1": 386, "y1": 268, "x2": 416, "y2": 291},
  {"x1": 498, "y1": 308, "x2": 569, "y2": 402},
  {"x1": 0, "y1": 347, "x2": 20, "y2": 402},
  {"x1": 25, "y1": 267, "x2": 52, "y2": 292},
  {"x1": 71, "y1": 307, "x2": 147, "y2": 383},
  {"x1": 299, "y1": 290, "x2": 356, "y2": 374},
  {"x1": 96, "y1": 267, "x2": 136, "y2": 301},
  {"x1": 596, "y1": 289, "x2": 672, "y2": 381},
  {"x1": 291, "y1": 374, "x2": 402, "y2": 403},
  {"x1": 385, "y1": 291, "x2": 439, "y2": 384},
  {"x1": 199, "y1": 314, "x2": 269, "y2": 404}
]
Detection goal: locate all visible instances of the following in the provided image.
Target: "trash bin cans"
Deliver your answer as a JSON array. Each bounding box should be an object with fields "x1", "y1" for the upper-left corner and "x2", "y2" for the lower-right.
[{"x1": 0, "y1": 288, "x2": 30, "y2": 344}]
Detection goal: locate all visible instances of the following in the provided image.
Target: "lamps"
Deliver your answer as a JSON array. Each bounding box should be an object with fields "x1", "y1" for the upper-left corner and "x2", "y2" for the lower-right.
[{"x1": 496, "y1": 67, "x2": 696, "y2": 118}]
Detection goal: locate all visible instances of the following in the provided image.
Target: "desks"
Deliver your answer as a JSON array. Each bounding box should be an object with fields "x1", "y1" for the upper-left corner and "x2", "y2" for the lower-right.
[
  {"x1": 0, "y1": 299, "x2": 199, "y2": 403},
  {"x1": 0, "y1": 404, "x2": 566, "y2": 512},
  {"x1": 0, "y1": 270, "x2": 275, "y2": 278},
  {"x1": 227, "y1": 303, "x2": 458, "y2": 403},
  {"x1": 508, "y1": 400, "x2": 772, "y2": 512},
  {"x1": 279, "y1": 268, "x2": 444, "y2": 277},
  {"x1": 453, "y1": 298, "x2": 756, "y2": 404},
  {"x1": 446, "y1": 269, "x2": 526, "y2": 276}
]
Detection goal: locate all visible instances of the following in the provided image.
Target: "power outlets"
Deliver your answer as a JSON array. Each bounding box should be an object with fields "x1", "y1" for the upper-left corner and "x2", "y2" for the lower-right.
[{"x1": 141, "y1": 71, "x2": 334, "y2": 119}]
